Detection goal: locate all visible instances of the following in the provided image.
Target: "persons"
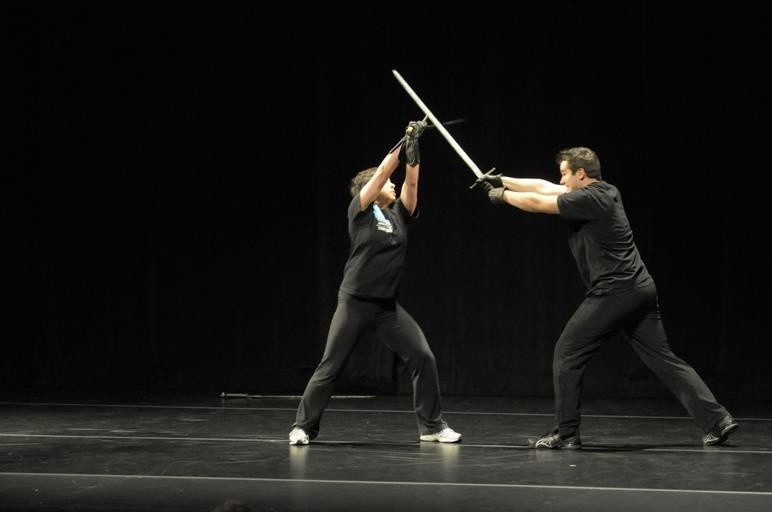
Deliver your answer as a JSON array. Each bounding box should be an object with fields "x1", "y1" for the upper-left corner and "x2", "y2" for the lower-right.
[
  {"x1": 476, "y1": 144, "x2": 741, "y2": 449},
  {"x1": 285, "y1": 119, "x2": 463, "y2": 446}
]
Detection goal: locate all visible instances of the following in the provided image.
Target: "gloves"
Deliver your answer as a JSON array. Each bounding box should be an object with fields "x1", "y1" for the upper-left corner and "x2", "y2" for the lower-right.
[
  {"x1": 390, "y1": 121, "x2": 428, "y2": 167},
  {"x1": 476, "y1": 173, "x2": 509, "y2": 207}
]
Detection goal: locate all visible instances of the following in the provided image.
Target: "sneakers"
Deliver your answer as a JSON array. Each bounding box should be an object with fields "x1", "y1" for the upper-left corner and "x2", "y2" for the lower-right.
[
  {"x1": 420, "y1": 427, "x2": 462, "y2": 443},
  {"x1": 288, "y1": 427, "x2": 310, "y2": 445},
  {"x1": 702, "y1": 413, "x2": 739, "y2": 447},
  {"x1": 535, "y1": 430, "x2": 582, "y2": 450}
]
